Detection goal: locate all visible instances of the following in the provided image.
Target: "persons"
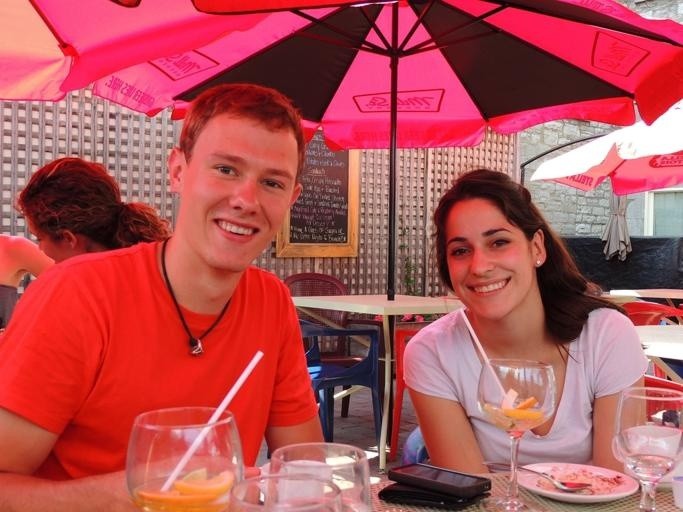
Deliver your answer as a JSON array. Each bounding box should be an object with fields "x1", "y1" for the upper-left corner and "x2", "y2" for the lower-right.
[
  {"x1": 0, "y1": 83, "x2": 329, "y2": 511},
  {"x1": 402, "y1": 168, "x2": 647, "y2": 474},
  {"x1": 15, "y1": 157, "x2": 171, "y2": 263},
  {"x1": 0, "y1": 234, "x2": 54, "y2": 327}
]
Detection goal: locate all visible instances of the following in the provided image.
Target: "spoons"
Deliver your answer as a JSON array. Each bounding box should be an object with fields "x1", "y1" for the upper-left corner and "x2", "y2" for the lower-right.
[{"x1": 480, "y1": 458, "x2": 591, "y2": 493}]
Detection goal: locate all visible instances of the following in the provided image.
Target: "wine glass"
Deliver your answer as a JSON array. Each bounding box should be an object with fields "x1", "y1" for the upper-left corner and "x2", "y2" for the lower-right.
[
  {"x1": 476, "y1": 358, "x2": 556, "y2": 511},
  {"x1": 611, "y1": 385, "x2": 683, "y2": 511}
]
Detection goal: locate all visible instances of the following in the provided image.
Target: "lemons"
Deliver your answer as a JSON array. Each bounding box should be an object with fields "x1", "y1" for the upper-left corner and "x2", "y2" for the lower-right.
[{"x1": 136, "y1": 471, "x2": 235, "y2": 510}]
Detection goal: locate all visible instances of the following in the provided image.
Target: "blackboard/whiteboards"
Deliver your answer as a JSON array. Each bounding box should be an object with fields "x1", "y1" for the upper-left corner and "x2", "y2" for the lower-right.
[
  {"x1": 275, "y1": 126, "x2": 361, "y2": 258},
  {"x1": 512, "y1": 114, "x2": 614, "y2": 246}
]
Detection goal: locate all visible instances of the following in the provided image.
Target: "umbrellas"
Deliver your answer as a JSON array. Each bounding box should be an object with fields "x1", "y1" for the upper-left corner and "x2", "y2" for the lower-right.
[
  {"x1": 531, "y1": 104, "x2": 682, "y2": 196},
  {"x1": 0, "y1": 1, "x2": 683, "y2": 446}
]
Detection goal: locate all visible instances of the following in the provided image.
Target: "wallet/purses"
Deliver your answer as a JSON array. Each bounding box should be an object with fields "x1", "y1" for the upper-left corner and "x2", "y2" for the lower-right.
[{"x1": 378, "y1": 483, "x2": 490, "y2": 511}]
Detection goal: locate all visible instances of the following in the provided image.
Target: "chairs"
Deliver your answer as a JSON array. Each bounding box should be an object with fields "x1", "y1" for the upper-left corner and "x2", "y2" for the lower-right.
[
  {"x1": 403, "y1": 426, "x2": 428, "y2": 465},
  {"x1": 283, "y1": 272, "x2": 385, "y2": 416},
  {"x1": 619, "y1": 300, "x2": 683, "y2": 426},
  {"x1": 298, "y1": 318, "x2": 393, "y2": 449},
  {"x1": 386, "y1": 323, "x2": 434, "y2": 462}
]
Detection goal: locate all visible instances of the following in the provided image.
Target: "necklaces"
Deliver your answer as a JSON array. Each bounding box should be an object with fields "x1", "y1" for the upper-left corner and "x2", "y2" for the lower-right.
[{"x1": 160, "y1": 237, "x2": 233, "y2": 355}]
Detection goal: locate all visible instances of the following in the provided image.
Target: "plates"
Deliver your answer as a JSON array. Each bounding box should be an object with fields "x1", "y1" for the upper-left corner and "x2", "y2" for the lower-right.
[{"x1": 516, "y1": 462, "x2": 639, "y2": 501}]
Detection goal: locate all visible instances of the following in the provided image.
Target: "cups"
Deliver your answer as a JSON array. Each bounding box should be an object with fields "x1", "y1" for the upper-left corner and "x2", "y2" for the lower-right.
[
  {"x1": 124, "y1": 405, "x2": 243, "y2": 511},
  {"x1": 268, "y1": 442, "x2": 372, "y2": 512},
  {"x1": 226, "y1": 473, "x2": 343, "y2": 511}
]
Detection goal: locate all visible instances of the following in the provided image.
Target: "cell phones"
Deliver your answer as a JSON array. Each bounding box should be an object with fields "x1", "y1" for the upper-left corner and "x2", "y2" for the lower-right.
[{"x1": 387, "y1": 462, "x2": 492, "y2": 499}]
